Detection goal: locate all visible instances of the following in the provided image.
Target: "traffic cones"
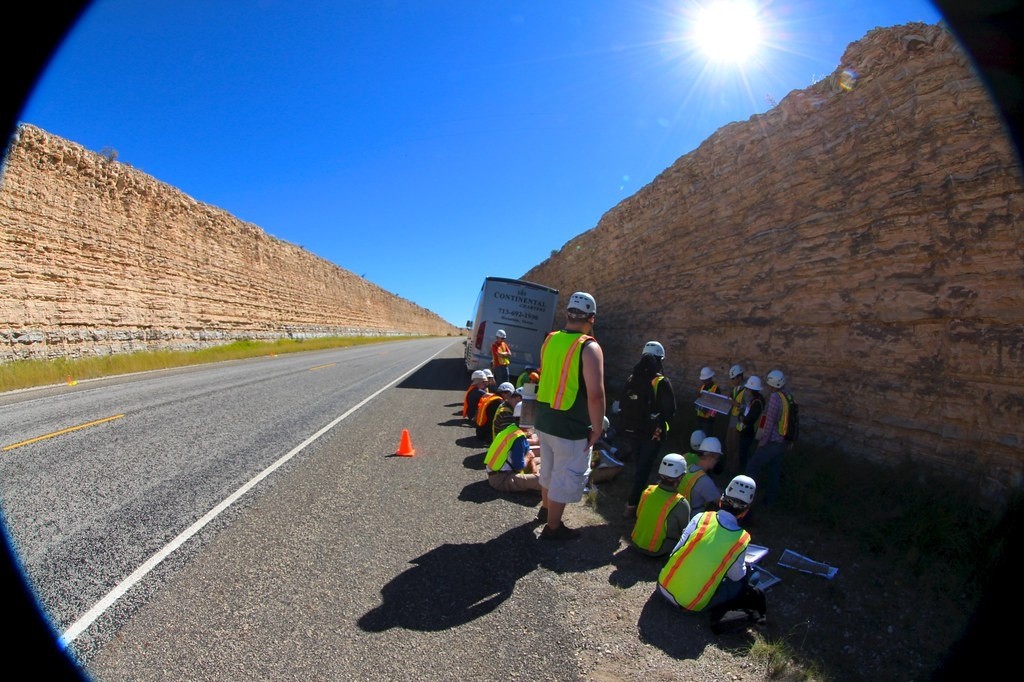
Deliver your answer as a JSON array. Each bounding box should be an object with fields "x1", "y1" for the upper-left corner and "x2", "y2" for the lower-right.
[{"x1": 395, "y1": 429, "x2": 417, "y2": 457}]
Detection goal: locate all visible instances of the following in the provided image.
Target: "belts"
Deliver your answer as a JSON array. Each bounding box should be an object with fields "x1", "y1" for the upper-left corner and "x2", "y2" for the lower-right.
[{"x1": 489, "y1": 471, "x2": 506, "y2": 475}]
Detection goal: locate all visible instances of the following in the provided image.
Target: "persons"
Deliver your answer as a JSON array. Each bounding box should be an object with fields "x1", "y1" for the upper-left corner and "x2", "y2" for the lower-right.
[
  {"x1": 725, "y1": 364, "x2": 746, "y2": 475},
  {"x1": 620, "y1": 341, "x2": 675, "y2": 521},
  {"x1": 484, "y1": 401, "x2": 542, "y2": 492},
  {"x1": 534, "y1": 292, "x2": 606, "y2": 539},
  {"x1": 630, "y1": 453, "x2": 691, "y2": 561},
  {"x1": 491, "y1": 329, "x2": 511, "y2": 385},
  {"x1": 476, "y1": 383, "x2": 515, "y2": 443},
  {"x1": 746, "y1": 370, "x2": 791, "y2": 497},
  {"x1": 656, "y1": 474, "x2": 759, "y2": 615},
  {"x1": 516, "y1": 364, "x2": 541, "y2": 394},
  {"x1": 695, "y1": 367, "x2": 721, "y2": 438},
  {"x1": 492, "y1": 387, "x2": 540, "y2": 457},
  {"x1": 738, "y1": 376, "x2": 765, "y2": 474},
  {"x1": 677, "y1": 437, "x2": 721, "y2": 520},
  {"x1": 588, "y1": 400, "x2": 620, "y2": 466},
  {"x1": 683, "y1": 430, "x2": 705, "y2": 464},
  {"x1": 483, "y1": 369, "x2": 498, "y2": 393},
  {"x1": 463, "y1": 370, "x2": 487, "y2": 429}
]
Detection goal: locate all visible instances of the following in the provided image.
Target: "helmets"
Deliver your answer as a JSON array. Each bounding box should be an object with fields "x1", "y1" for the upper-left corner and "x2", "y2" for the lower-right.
[
  {"x1": 698, "y1": 436, "x2": 723, "y2": 455},
  {"x1": 471, "y1": 370, "x2": 486, "y2": 379},
  {"x1": 528, "y1": 372, "x2": 539, "y2": 380},
  {"x1": 766, "y1": 370, "x2": 785, "y2": 389},
  {"x1": 567, "y1": 291, "x2": 596, "y2": 314},
  {"x1": 699, "y1": 367, "x2": 715, "y2": 379},
  {"x1": 513, "y1": 401, "x2": 523, "y2": 417},
  {"x1": 515, "y1": 387, "x2": 522, "y2": 396},
  {"x1": 725, "y1": 474, "x2": 756, "y2": 504},
  {"x1": 497, "y1": 382, "x2": 516, "y2": 395},
  {"x1": 641, "y1": 340, "x2": 666, "y2": 361},
  {"x1": 602, "y1": 417, "x2": 611, "y2": 433},
  {"x1": 483, "y1": 369, "x2": 493, "y2": 377},
  {"x1": 690, "y1": 430, "x2": 704, "y2": 451},
  {"x1": 659, "y1": 453, "x2": 688, "y2": 478},
  {"x1": 744, "y1": 376, "x2": 764, "y2": 390},
  {"x1": 524, "y1": 365, "x2": 535, "y2": 370},
  {"x1": 496, "y1": 329, "x2": 506, "y2": 338},
  {"x1": 729, "y1": 365, "x2": 744, "y2": 379}
]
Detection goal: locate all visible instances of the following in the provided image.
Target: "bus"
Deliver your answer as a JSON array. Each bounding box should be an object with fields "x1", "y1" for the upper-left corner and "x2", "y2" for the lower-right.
[{"x1": 464, "y1": 276, "x2": 559, "y2": 377}]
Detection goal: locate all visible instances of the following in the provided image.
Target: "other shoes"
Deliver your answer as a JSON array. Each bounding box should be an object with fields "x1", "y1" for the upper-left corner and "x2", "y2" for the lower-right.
[
  {"x1": 623, "y1": 502, "x2": 637, "y2": 516},
  {"x1": 538, "y1": 507, "x2": 550, "y2": 522},
  {"x1": 720, "y1": 610, "x2": 748, "y2": 622},
  {"x1": 542, "y1": 520, "x2": 581, "y2": 540}
]
